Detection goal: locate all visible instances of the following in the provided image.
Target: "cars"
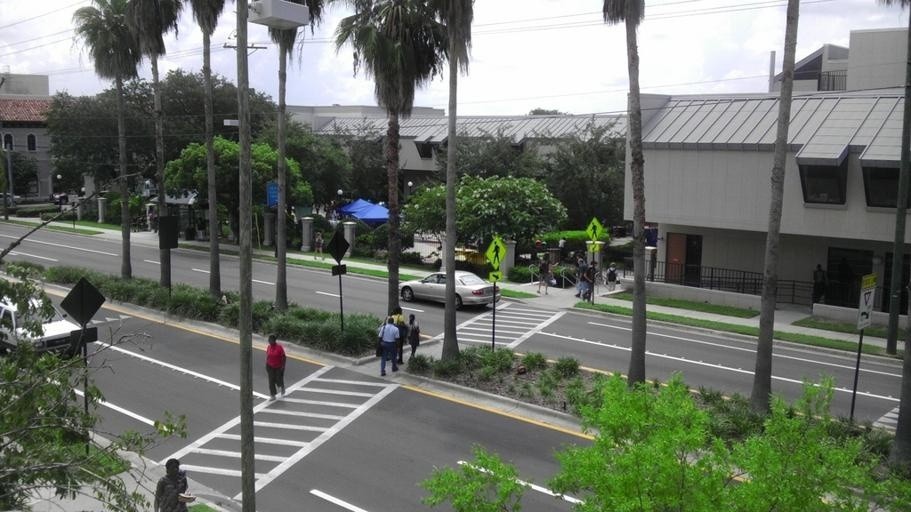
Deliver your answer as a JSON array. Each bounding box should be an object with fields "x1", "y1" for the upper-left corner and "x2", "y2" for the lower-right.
[
  {"x1": 398, "y1": 269, "x2": 502, "y2": 310},
  {"x1": 0, "y1": 295, "x2": 82, "y2": 355}
]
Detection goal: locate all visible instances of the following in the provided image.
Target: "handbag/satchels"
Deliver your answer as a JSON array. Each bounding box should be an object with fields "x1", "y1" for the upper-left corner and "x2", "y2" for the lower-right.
[
  {"x1": 375, "y1": 344, "x2": 382, "y2": 357},
  {"x1": 395, "y1": 325, "x2": 407, "y2": 338},
  {"x1": 177, "y1": 490, "x2": 196, "y2": 503}
]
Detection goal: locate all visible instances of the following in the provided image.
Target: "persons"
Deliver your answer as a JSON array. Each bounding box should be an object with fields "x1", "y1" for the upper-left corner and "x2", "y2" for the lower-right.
[
  {"x1": 809, "y1": 262, "x2": 826, "y2": 305},
  {"x1": 313, "y1": 231, "x2": 325, "y2": 260},
  {"x1": 377, "y1": 316, "x2": 402, "y2": 375},
  {"x1": 153, "y1": 457, "x2": 189, "y2": 511},
  {"x1": 264, "y1": 333, "x2": 286, "y2": 402},
  {"x1": 528, "y1": 235, "x2": 619, "y2": 303},
  {"x1": 390, "y1": 307, "x2": 408, "y2": 365},
  {"x1": 130, "y1": 211, "x2": 160, "y2": 234},
  {"x1": 405, "y1": 314, "x2": 420, "y2": 358},
  {"x1": 195, "y1": 217, "x2": 205, "y2": 241},
  {"x1": 381, "y1": 311, "x2": 394, "y2": 362}
]
